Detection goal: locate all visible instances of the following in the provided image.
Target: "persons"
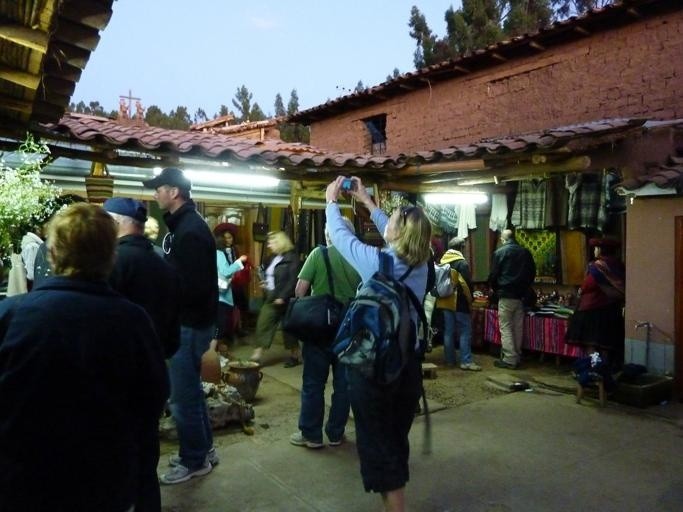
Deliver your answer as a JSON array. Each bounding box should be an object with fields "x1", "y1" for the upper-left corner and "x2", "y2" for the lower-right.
[
  {"x1": 143, "y1": 215, "x2": 166, "y2": 260},
  {"x1": 21, "y1": 221, "x2": 45, "y2": 293},
  {"x1": 289, "y1": 216, "x2": 362, "y2": 448},
  {"x1": 143, "y1": 167, "x2": 220, "y2": 485},
  {"x1": 103, "y1": 196, "x2": 182, "y2": 359},
  {"x1": 565, "y1": 239, "x2": 625, "y2": 397},
  {"x1": 435, "y1": 237, "x2": 484, "y2": 370},
  {"x1": 246, "y1": 229, "x2": 303, "y2": 369},
  {"x1": 487, "y1": 229, "x2": 536, "y2": 370},
  {"x1": 209, "y1": 230, "x2": 248, "y2": 346},
  {"x1": 324, "y1": 175, "x2": 437, "y2": 512},
  {"x1": 0, "y1": 201, "x2": 170, "y2": 511},
  {"x1": 214, "y1": 223, "x2": 253, "y2": 340}
]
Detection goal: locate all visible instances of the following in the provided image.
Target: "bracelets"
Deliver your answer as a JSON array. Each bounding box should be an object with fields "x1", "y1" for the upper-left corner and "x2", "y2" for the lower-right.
[{"x1": 327, "y1": 200, "x2": 336, "y2": 207}]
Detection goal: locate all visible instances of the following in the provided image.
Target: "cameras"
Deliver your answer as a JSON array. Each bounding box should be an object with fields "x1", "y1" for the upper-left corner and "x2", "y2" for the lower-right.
[{"x1": 340, "y1": 178, "x2": 354, "y2": 190}]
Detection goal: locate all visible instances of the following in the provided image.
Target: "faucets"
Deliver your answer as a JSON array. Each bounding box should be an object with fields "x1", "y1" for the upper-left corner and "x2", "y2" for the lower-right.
[{"x1": 635, "y1": 322, "x2": 647, "y2": 330}]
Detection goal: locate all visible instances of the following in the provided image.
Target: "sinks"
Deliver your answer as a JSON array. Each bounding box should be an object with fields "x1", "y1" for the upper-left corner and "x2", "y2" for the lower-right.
[{"x1": 611, "y1": 370, "x2": 674, "y2": 410}]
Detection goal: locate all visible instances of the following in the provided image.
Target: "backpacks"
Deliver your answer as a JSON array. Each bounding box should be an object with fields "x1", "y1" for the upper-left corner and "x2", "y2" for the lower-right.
[
  {"x1": 332, "y1": 271, "x2": 421, "y2": 387},
  {"x1": 430, "y1": 262, "x2": 456, "y2": 298}
]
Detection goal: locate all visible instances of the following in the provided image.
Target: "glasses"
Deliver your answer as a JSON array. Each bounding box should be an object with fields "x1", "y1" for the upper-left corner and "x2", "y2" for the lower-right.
[{"x1": 399, "y1": 204, "x2": 419, "y2": 224}]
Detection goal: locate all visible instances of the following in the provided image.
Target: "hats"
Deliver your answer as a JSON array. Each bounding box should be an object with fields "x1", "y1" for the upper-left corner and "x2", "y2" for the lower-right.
[
  {"x1": 102, "y1": 195, "x2": 148, "y2": 222},
  {"x1": 587, "y1": 236, "x2": 618, "y2": 249},
  {"x1": 142, "y1": 167, "x2": 191, "y2": 188},
  {"x1": 213, "y1": 223, "x2": 240, "y2": 235}
]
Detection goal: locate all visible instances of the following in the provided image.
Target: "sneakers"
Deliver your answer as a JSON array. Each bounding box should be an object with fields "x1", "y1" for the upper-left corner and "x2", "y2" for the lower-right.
[
  {"x1": 494, "y1": 358, "x2": 518, "y2": 370},
  {"x1": 284, "y1": 355, "x2": 300, "y2": 367},
  {"x1": 159, "y1": 456, "x2": 212, "y2": 484},
  {"x1": 247, "y1": 358, "x2": 263, "y2": 367},
  {"x1": 289, "y1": 430, "x2": 323, "y2": 448},
  {"x1": 326, "y1": 432, "x2": 344, "y2": 446},
  {"x1": 168, "y1": 446, "x2": 220, "y2": 465},
  {"x1": 459, "y1": 360, "x2": 483, "y2": 371},
  {"x1": 445, "y1": 361, "x2": 456, "y2": 368}
]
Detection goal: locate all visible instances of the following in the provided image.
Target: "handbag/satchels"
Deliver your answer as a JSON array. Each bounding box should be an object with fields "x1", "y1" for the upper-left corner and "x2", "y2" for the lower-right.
[
  {"x1": 250, "y1": 221, "x2": 270, "y2": 242},
  {"x1": 217, "y1": 271, "x2": 231, "y2": 292},
  {"x1": 278, "y1": 290, "x2": 344, "y2": 340},
  {"x1": 521, "y1": 284, "x2": 537, "y2": 306}
]
